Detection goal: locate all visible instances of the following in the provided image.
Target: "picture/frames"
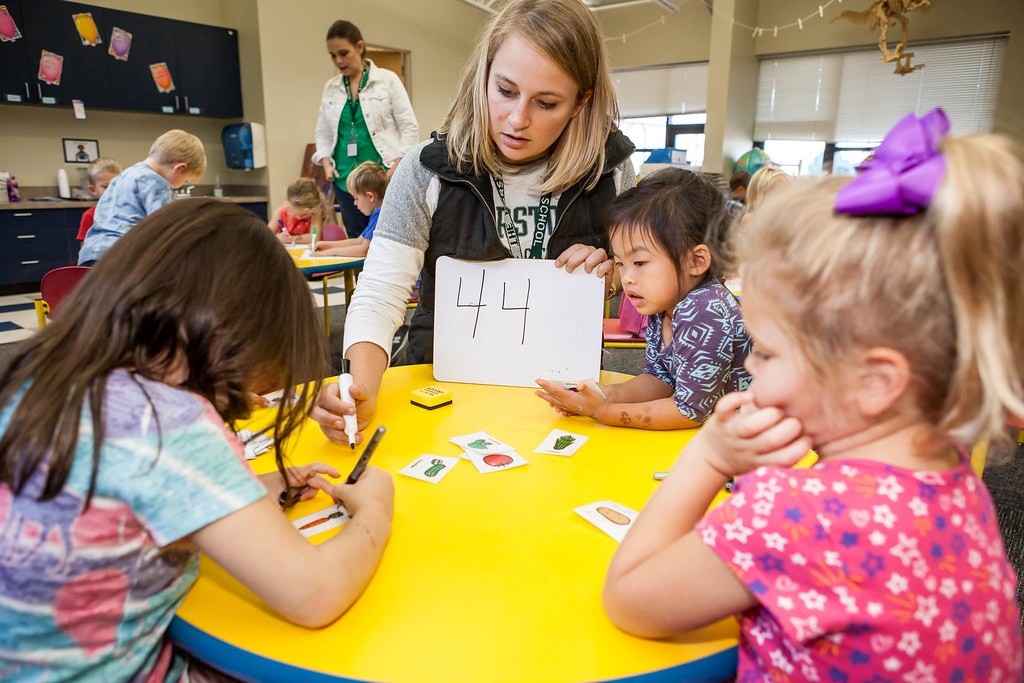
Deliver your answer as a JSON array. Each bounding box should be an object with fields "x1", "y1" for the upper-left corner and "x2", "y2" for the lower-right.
[{"x1": 62, "y1": 138, "x2": 99, "y2": 164}]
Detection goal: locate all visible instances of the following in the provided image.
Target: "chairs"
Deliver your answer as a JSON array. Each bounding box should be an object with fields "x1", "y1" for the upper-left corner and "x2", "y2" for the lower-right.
[
  {"x1": 307, "y1": 224, "x2": 354, "y2": 338},
  {"x1": 34, "y1": 267, "x2": 97, "y2": 330}
]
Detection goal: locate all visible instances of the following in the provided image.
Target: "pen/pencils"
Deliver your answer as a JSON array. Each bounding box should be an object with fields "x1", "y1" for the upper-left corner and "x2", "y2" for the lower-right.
[
  {"x1": 653, "y1": 472, "x2": 668, "y2": 479},
  {"x1": 337, "y1": 426, "x2": 388, "y2": 507}
]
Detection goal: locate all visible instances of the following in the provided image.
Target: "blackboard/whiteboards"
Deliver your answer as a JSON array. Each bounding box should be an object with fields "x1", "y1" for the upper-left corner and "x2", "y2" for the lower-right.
[{"x1": 432, "y1": 255, "x2": 605, "y2": 388}]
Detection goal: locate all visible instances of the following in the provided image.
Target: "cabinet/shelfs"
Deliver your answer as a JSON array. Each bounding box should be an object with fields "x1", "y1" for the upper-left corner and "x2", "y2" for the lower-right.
[
  {"x1": 0, "y1": 197, "x2": 96, "y2": 285},
  {"x1": 0, "y1": 0, "x2": 112, "y2": 110},
  {"x1": 103, "y1": 7, "x2": 243, "y2": 117}
]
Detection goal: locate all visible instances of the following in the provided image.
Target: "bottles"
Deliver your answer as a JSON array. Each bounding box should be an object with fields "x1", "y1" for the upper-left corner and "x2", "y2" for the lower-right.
[
  {"x1": 57, "y1": 169, "x2": 71, "y2": 199},
  {"x1": 8, "y1": 176, "x2": 20, "y2": 202},
  {"x1": 214, "y1": 175, "x2": 223, "y2": 197}
]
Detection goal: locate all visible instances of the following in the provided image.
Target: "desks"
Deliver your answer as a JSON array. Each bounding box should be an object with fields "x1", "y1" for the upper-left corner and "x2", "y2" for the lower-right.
[
  {"x1": 283, "y1": 243, "x2": 366, "y2": 313},
  {"x1": 170, "y1": 364, "x2": 819, "y2": 683}
]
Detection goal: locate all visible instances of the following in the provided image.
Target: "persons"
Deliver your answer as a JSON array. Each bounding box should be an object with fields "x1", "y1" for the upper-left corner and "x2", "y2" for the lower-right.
[
  {"x1": 534, "y1": 166, "x2": 753, "y2": 432},
  {"x1": 266, "y1": 177, "x2": 336, "y2": 245},
  {"x1": 1, "y1": 197, "x2": 393, "y2": 683},
  {"x1": 78, "y1": 129, "x2": 207, "y2": 269},
  {"x1": 75, "y1": 159, "x2": 122, "y2": 251},
  {"x1": 601, "y1": 106, "x2": 1024, "y2": 682},
  {"x1": 313, "y1": 160, "x2": 409, "y2": 368},
  {"x1": 723, "y1": 171, "x2": 753, "y2": 220},
  {"x1": 311, "y1": 20, "x2": 420, "y2": 281},
  {"x1": 309, "y1": 0, "x2": 637, "y2": 446}
]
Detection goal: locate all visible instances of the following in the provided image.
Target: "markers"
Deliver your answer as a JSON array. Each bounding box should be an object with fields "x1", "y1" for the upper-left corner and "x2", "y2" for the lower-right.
[
  {"x1": 337, "y1": 359, "x2": 358, "y2": 450},
  {"x1": 311, "y1": 227, "x2": 317, "y2": 255},
  {"x1": 277, "y1": 220, "x2": 296, "y2": 246}
]
